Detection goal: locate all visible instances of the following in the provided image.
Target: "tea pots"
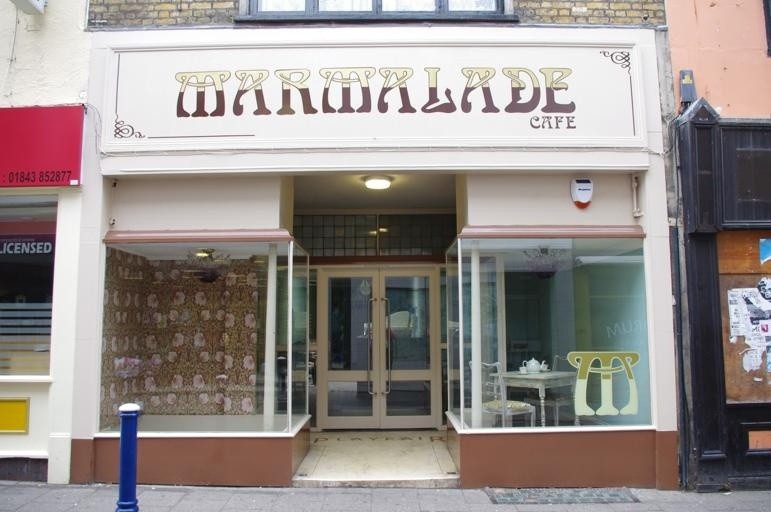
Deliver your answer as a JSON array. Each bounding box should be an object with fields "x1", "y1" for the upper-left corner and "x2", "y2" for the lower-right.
[{"x1": 522, "y1": 357, "x2": 547, "y2": 373}]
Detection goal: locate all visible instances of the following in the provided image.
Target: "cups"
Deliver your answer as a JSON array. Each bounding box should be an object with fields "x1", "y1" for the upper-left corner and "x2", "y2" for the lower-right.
[
  {"x1": 520, "y1": 366, "x2": 529, "y2": 374},
  {"x1": 541, "y1": 364, "x2": 549, "y2": 369}
]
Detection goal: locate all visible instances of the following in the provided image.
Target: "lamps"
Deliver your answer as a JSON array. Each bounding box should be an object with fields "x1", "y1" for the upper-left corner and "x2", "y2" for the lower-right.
[
  {"x1": 363, "y1": 173, "x2": 394, "y2": 190},
  {"x1": 194, "y1": 248, "x2": 210, "y2": 259}
]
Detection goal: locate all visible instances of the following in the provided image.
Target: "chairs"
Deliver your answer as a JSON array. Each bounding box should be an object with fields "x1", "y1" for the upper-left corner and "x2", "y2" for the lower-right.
[{"x1": 468, "y1": 354, "x2": 582, "y2": 429}]
